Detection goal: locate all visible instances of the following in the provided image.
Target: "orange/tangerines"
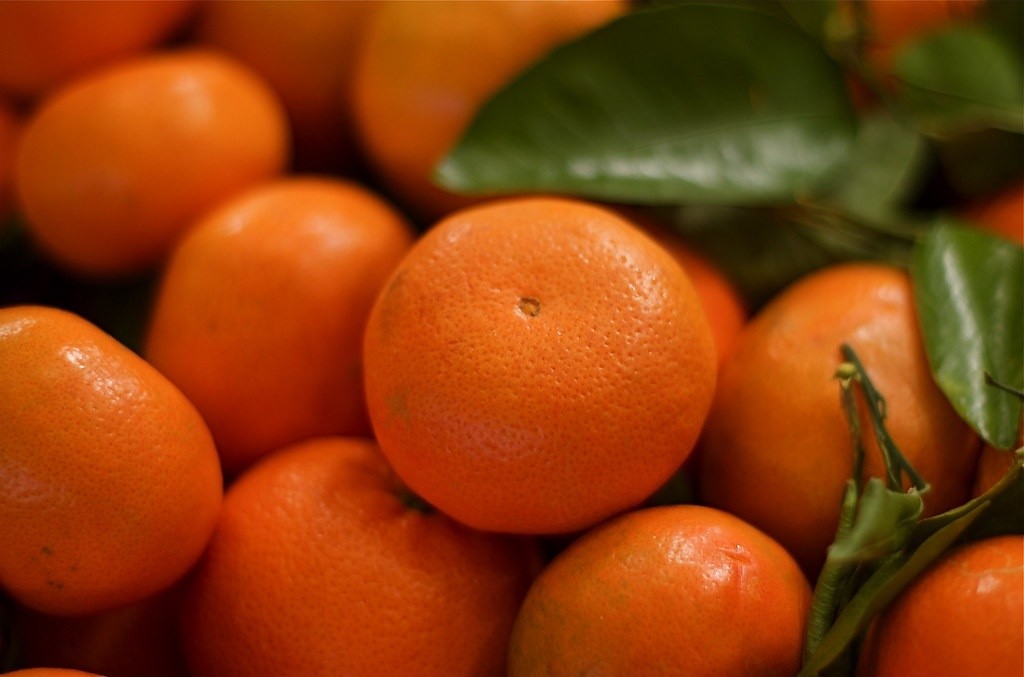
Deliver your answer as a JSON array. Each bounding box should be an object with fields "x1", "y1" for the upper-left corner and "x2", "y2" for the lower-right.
[{"x1": 0, "y1": 0, "x2": 1024, "y2": 677}]
